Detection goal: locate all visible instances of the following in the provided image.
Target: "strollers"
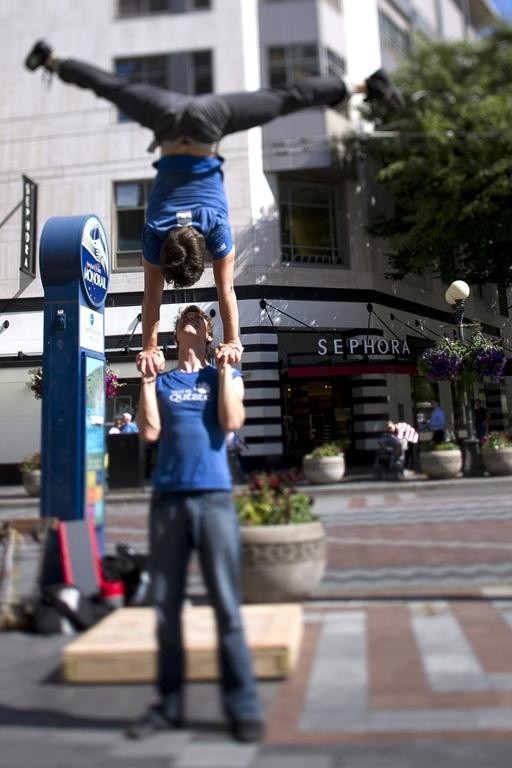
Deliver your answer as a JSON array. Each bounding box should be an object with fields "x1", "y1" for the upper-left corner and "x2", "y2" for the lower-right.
[{"x1": 372, "y1": 433, "x2": 404, "y2": 481}]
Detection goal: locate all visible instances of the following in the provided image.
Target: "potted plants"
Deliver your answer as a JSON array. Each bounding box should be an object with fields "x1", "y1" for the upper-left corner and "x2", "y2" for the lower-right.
[
  {"x1": 20, "y1": 452, "x2": 41, "y2": 497},
  {"x1": 419, "y1": 442, "x2": 462, "y2": 478},
  {"x1": 304, "y1": 442, "x2": 347, "y2": 485}
]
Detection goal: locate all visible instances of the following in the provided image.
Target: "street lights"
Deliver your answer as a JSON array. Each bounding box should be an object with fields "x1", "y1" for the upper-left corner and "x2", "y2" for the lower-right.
[{"x1": 444, "y1": 280, "x2": 485, "y2": 478}]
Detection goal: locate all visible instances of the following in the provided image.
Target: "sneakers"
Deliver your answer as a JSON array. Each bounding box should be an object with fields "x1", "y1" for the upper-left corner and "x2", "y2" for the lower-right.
[
  {"x1": 127, "y1": 706, "x2": 172, "y2": 737},
  {"x1": 367, "y1": 68, "x2": 406, "y2": 110},
  {"x1": 25, "y1": 39, "x2": 52, "y2": 71},
  {"x1": 230, "y1": 719, "x2": 264, "y2": 742}
]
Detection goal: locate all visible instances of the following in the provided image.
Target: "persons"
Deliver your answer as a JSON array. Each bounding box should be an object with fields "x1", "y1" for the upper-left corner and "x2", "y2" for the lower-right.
[
  {"x1": 385, "y1": 420, "x2": 421, "y2": 477},
  {"x1": 119, "y1": 412, "x2": 139, "y2": 434},
  {"x1": 233, "y1": 426, "x2": 252, "y2": 479},
  {"x1": 420, "y1": 396, "x2": 446, "y2": 446},
  {"x1": 471, "y1": 398, "x2": 490, "y2": 439},
  {"x1": 108, "y1": 418, "x2": 123, "y2": 435},
  {"x1": 442, "y1": 420, "x2": 459, "y2": 445},
  {"x1": 125, "y1": 303, "x2": 266, "y2": 744},
  {"x1": 21, "y1": 34, "x2": 409, "y2": 377},
  {"x1": 225, "y1": 429, "x2": 251, "y2": 483}
]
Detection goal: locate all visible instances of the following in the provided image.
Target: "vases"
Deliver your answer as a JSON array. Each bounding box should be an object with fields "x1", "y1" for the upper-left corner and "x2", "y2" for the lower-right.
[
  {"x1": 239, "y1": 517, "x2": 327, "y2": 602},
  {"x1": 480, "y1": 448, "x2": 512, "y2": 476}
]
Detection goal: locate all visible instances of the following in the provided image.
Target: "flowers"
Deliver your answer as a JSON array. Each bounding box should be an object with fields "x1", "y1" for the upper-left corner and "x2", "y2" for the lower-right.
[
  {"x1": 234, "y1": 468, "x2": 316, "y2": 524},
  {"x1": 481, "y1": 431, "x2": 506, "y2": 450}
]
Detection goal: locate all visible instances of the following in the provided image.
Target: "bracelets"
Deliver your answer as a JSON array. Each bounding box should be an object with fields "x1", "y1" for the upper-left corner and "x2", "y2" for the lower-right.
[{"x1": 139, "y1": 377, "x2": 158, "y2": 386}]
